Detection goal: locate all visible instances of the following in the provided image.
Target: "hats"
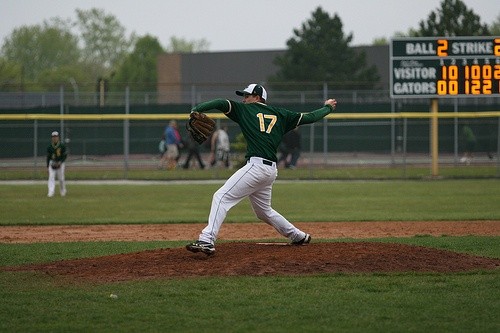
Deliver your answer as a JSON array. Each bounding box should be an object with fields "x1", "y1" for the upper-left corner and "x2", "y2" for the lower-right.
[
  {"x1": 51, "y1": 132, "x2": 58, "y2": 136},
  {"x1": 235, "y1": 83, "x2": 266, "y2": 100}
]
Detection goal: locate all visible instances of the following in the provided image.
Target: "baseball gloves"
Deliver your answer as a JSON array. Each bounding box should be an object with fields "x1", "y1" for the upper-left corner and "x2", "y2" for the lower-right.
[
  {"x1": 52, "y1": 162, "x2": 61, "y2": 169},
  {"x1": 187, "y1": 112, "x2": 216, "y2": 143}
]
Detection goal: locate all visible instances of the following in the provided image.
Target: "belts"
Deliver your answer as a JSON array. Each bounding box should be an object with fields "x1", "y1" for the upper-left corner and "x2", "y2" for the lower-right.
[{"x1": 248, "y1": 158, "x2": 276, "y2": 167}]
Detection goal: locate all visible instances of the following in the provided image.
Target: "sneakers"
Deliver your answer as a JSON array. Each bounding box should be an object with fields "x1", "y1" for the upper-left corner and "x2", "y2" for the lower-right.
[
  {"x1": 290, "y1": 233, "x2": 311, "y2": 243},
  {"x1": 186, "y1": 241, "x2": 216, "y2": 254}
]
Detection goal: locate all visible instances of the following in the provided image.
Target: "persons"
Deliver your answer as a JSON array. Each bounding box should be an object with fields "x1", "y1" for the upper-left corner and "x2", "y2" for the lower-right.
[
  {"x1": 277, "y1": 127, "x2": 301, "y2": 169},
  {"x1": 209, "y1": 125, "x2": 230, "y2": 169},
  {"x1": 159, "y1": 120, "x2": 205, "y2": 169},
  {"x1": 185, "y1": 83, "x2": 337, "y2": 255},
  {"x1": 46, "y1": 131, "x2": 67, "y2": 197}
]
